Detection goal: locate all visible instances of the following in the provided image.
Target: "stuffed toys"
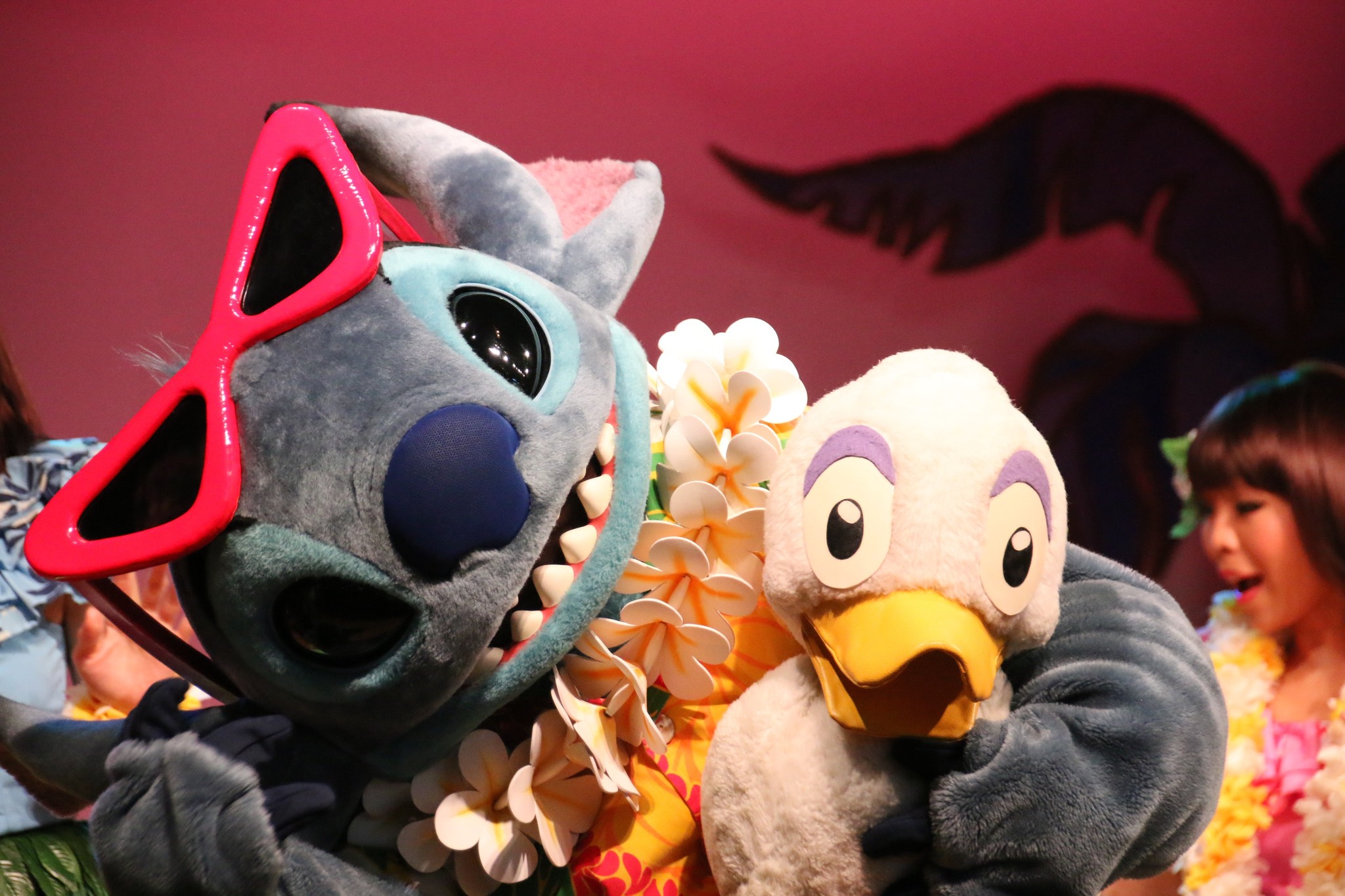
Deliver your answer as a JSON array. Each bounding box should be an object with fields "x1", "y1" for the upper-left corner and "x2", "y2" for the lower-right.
[{"x1": 0, "y1": 102, "x2": 1227, "y2": 896}]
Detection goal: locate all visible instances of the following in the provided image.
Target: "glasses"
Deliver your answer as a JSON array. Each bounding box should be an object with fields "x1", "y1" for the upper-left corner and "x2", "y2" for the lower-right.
[{"x1": 24, "y1": 105, "x2": 425, "y2": 704}]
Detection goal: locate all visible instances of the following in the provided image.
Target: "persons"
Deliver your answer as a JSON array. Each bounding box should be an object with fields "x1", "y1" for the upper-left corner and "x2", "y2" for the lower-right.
[
  {"x1": 0, "y1": 338, "x2": 194, "y2": 835},
  {"x1": 1099, "y1": 357, "x2": 1345, "y2": 896}
]
